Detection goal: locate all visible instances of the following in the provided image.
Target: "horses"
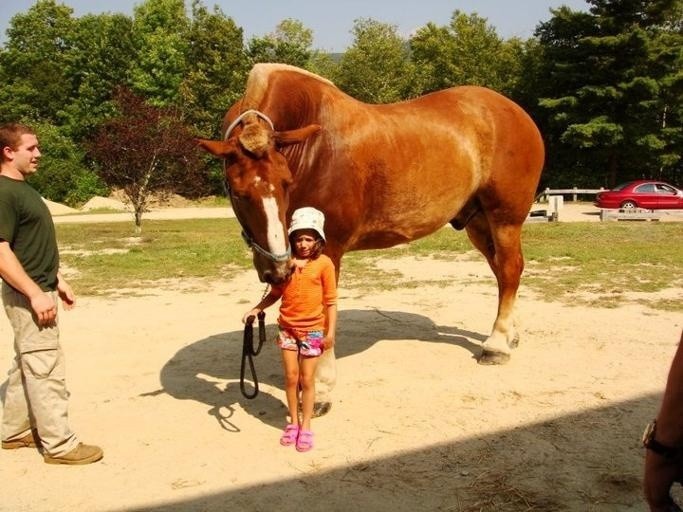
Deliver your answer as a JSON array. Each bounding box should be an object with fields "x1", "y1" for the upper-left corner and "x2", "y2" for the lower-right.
[{"x1": 195, "y1": 59, "x2": 551, "y2": 421}]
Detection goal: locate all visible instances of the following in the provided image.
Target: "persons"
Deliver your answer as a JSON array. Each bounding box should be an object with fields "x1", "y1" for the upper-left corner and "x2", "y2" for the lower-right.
[
  {"x1": 642, "y1": 327, "x2": 683, "y2": 512},
  {"x1": 240, "y1": 204, "x2": 338, "y2": 453},
  {"x1": 1, "y1": 120, "x2": 106, "y2": 466}
]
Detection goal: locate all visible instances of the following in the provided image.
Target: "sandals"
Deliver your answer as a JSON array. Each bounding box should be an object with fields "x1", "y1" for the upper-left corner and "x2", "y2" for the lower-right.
[
  {"x1": 280, "y1": 423, "x2": 299, "y2": 447},
  {"x1": 296, "y1": 431, "x2": 313, "y2": 452}
]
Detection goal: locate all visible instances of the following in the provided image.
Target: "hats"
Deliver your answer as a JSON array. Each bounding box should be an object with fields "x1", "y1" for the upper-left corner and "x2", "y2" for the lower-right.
[{"x1": 287, "y1": 206, "x2": 327, "y2": 243}]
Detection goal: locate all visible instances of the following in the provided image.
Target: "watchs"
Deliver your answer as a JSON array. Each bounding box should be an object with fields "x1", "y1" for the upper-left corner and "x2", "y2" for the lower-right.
[{"x1": 640, "y1": 417, "x2": 671, "y2": 456}]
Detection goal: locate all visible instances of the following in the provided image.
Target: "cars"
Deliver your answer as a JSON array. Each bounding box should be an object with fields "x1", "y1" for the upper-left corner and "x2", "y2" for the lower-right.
[{"x1": 596, "y1": 180, "x2": 682, "y2": 209}]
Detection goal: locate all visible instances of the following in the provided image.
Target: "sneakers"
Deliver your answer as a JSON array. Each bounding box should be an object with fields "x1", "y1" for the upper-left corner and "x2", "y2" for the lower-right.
[
  {"x1": 1, "y1": 428, "x2": 42, "y2": 449},
  {"x1": 42, "y1": 442, "x2": 103, "y2": 465}
]
